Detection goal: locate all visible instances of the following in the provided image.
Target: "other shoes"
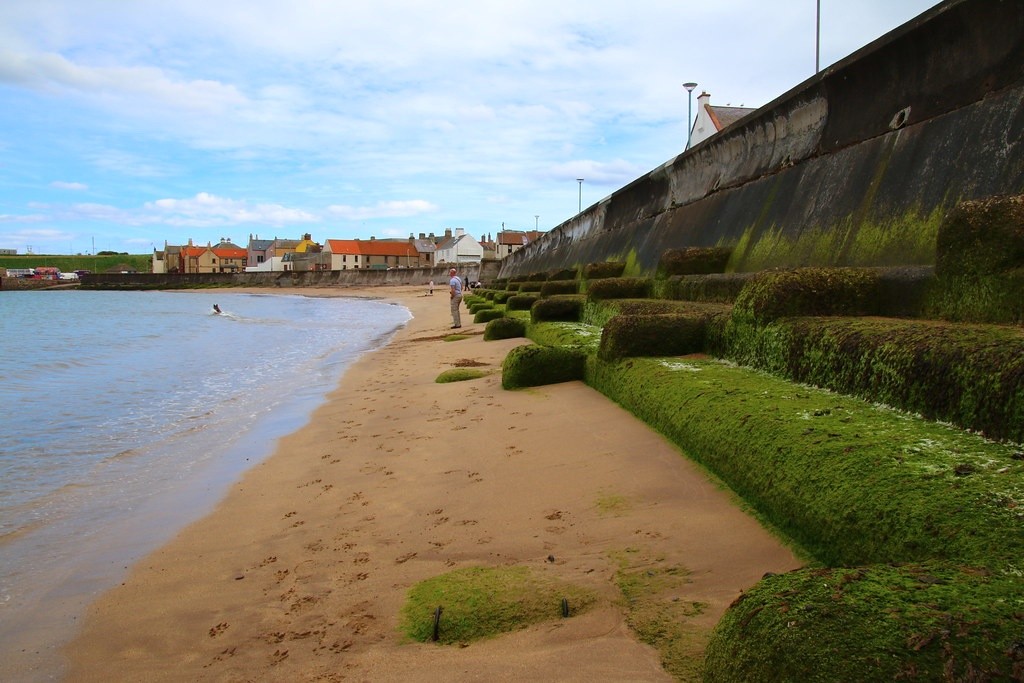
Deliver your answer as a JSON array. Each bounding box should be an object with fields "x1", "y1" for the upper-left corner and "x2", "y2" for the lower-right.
[{"x1": 451, "y1": 326, "x2": 460, "y2": 329}]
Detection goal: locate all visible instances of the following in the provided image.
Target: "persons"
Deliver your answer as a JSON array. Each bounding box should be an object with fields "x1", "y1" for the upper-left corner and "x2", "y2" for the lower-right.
[
  {"x1": 449, "y1": 268, "x2": 462, "y2": 329},
  {"x1": 463, "y1": 276, "x2": 483, "y2": 291},
  {"x1": 429, "y1": 279, "x2": 434, "y2": 296}
]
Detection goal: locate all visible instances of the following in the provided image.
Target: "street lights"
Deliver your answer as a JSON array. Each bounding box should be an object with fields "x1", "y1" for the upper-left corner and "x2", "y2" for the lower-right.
[
  {"x1": 576, "y1": 179, "x2": 584, "y2": 214},
  {"x1": 684, "y1": 82, "x2": 698, "y2": 149},
  {"x1": 535, "y1": 216, "x2": 539, "y2": 240}
]
